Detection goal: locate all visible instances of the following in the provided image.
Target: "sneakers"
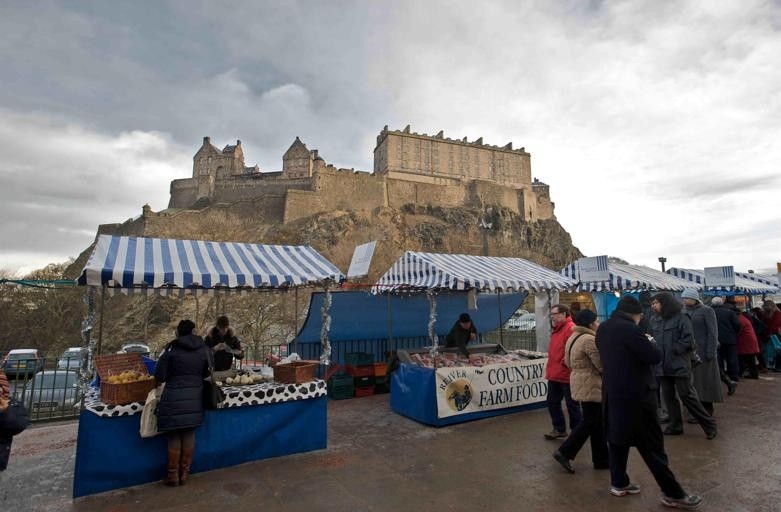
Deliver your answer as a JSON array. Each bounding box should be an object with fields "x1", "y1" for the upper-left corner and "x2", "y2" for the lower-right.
[
  {"x1": 544, "y1": 428, "x2": 567, "y2": 438},
  {"x1": 660, "y1": 492, "x2": 703, "y2": 508},
  {"x1": 554, "y1": 448, "x2": 576, "y2": 473},
  {"x1": 661, "y1": 367, "x2": 779, "y2": 440},
  {"x1": 609, "y1": 483, "x2": 644, "y2": 497}
]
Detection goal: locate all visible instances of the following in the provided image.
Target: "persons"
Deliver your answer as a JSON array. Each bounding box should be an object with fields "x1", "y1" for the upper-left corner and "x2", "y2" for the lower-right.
[
  {"x1": 446, "y1": 313, "x2": 479, "y2": 357},
  {"x1": 0, "y1": 369, "x2": 13, "y2": 470},
  {"x1": 203, "y1": 314, "x2": 244, "y2": 370},
  {"x1": 155, "y1": 317, "x2": 210, "y2": 486}
]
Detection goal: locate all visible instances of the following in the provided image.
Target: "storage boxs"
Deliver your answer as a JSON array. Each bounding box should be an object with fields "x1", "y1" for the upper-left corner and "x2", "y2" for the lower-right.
[{"x1": 324, "y1": 350, "x2": 391, "y2": 401}]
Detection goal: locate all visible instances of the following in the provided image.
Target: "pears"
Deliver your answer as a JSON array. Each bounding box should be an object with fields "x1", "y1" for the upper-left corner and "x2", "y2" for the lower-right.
[
  {"x1": 217, "y1": 374, "x2": 262, "y2": 387},
  {"x1": 109, "y1": 371, "x2": 154, "y2": 384}
]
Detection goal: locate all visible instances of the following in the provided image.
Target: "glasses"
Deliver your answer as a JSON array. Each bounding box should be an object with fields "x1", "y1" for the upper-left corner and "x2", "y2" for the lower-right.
[{"x1": 549, "y1": 311, "x2": 562, "y2": 315}]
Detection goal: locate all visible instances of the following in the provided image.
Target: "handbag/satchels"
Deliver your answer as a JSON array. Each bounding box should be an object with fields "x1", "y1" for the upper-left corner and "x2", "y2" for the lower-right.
[
  {"x1": 140, "y1": 383, "x2": 167, "y2": 437},
  {"x1": 1, "y1": 399, "x2": 29, "y2": 434},
  {"x1": 203, "y1": 376, "x2": 227, "y2": 404}
]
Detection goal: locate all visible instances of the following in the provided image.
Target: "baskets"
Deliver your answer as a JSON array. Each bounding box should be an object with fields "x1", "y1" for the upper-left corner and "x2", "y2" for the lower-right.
[
  {"x1": 99, "y1": 375, "x2": 157, "y2": 406},
  {"x1": 273, "y1": 362, "x2": 315, "y2": 383}
]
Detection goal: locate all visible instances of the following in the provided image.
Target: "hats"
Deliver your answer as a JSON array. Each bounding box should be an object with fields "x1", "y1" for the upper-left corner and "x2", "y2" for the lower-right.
[
  {"x1": 711, "y1": 296, "x2": 724, "y2": 307},
  {"x1": 574, "y1": 310, "x2": 596, "y2": 325},
  {"x1": 617, "y1": 296, "x2": 643, "y2": 315},
  {"x1": 681, "y1": 289, "x2": 700, "y2": 300}
]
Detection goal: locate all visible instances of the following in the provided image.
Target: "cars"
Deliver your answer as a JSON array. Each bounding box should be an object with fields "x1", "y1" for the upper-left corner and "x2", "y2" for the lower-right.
[
  {"x1": 265, "y1": 348, "x2": 286, "y2": 364},
  {"x1": 53, "y1": 345, "x2": 93, "y2": 372},
  {"x1": 17, "y1": 369, "x2": 80, "y2": 416},
  {"x1": 118, "y1": 342, "x2": 150, "y2": 358},
  {"x1": 506, "y1": 307, "x2": 535, "y2": 331},
  {"x1": 2, "y1": 348, "x2": 45, "y2": 379}
]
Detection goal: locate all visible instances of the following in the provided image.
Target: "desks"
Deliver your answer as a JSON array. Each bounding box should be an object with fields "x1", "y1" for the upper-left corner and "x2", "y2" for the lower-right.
[
  {"x1": 78, "y1": 381, "x2": 326, "y2": 495},
  {"x1": 390, "y1": 352, "x2": 551, "y2": 426}
]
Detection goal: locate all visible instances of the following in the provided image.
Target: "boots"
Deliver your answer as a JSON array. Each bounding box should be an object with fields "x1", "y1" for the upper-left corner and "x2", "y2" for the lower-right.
[
  {"x1": 180, "y1": 444, "x2": 195, "y2": 485},
  {"x1": 162, "y1": 447, "x2": 178, "y2": 487}
]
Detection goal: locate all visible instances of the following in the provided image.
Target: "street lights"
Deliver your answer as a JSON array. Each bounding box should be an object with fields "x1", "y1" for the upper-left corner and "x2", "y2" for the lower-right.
[
  {"x1": 477, "y1": 217, "x2": 493, "y2": 255},
  {"x1": 657, "y1": 256, "x2": 667, "y2": 274}
]
